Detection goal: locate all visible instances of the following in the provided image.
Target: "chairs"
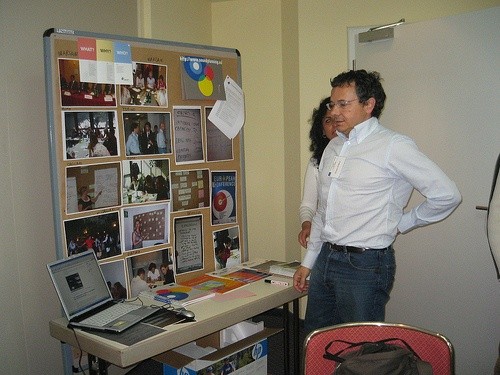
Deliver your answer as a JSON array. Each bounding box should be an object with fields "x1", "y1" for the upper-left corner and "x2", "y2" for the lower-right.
[{"x1": 301, "y1": 320, "x2": 456, "y2": 375}]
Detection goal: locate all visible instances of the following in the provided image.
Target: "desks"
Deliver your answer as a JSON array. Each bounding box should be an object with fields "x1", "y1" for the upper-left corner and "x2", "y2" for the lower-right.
[{"x1": 48, "y1": 256, "x2": 312, "y2": 375}]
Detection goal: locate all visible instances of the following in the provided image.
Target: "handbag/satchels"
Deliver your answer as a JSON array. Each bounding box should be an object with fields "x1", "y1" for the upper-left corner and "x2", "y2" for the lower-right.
[{"x1": 323, "y1": 337, "x2": 433, "y2": 375}]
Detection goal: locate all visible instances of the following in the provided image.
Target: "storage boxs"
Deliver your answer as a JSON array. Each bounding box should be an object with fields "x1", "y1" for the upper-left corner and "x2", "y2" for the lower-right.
[{"x1": 150, "y1": 320, "x2": 285, "y2": 375}]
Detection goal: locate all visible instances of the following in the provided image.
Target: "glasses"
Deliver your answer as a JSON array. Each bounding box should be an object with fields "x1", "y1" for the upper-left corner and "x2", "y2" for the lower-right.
[{"x1": 325, "y1": 96, "x2": 377, "y2": 111}]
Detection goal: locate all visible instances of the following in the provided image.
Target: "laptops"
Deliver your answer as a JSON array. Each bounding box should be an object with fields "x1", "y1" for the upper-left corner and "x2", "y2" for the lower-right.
[{"x1": 46, "y1": 249, "x2": 160, "y2": 333}]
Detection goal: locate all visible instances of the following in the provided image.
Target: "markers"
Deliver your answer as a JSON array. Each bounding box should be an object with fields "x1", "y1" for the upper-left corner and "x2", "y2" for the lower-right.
[{"x1": 264, "y1": 279, "x2": 289, "y2": 286}]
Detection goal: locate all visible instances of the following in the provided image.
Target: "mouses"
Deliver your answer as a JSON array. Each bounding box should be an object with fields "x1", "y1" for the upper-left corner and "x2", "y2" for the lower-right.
[{"x1": 180, "y1": 310, "x2": 195, "y2": 320}]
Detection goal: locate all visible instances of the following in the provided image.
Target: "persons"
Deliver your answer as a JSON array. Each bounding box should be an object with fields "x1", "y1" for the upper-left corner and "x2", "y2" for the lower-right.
[
  {"x1": 292, "y1": 69, "x2": 462, "y2": 330},
  {"x1": 77, "y1": 186, "x2": 97, "y2": 212},
  {"x1": 130, "y1": 262, "x2": 175, "y2": 298},
  {"x1": 70, "y1": 126, "x2": 117, "y2": 157},
  {"x1": 68, "y1": 233, "x2": 122, "y2": 260},
  {"x1": 121, "y1": 70, "x2": 166, "y2": 90},
  {"x1": 132, "y1": 220, "x2": 145, "y2": 249},
  {"x1": 130, "y1": 173, "x2": 169, "y2": 200},
  {"x1": 214, "y1": 229, "x2": 231, "y2": 269},
  {"x1": 107, "y1": 281, "x2": 127, "y2": 299},
  {"x1": 296, "y1": 94, "x2": 337, "y2": 249},
  {"x1": 126, "y1": 121, "x2": 169, "y2": 156},
  {"x1": 60, "y1": 75, "x2": 115, "y2": 93}
]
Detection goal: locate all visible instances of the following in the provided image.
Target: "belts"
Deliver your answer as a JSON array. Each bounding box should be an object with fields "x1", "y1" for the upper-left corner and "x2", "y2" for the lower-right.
[{"x1": 325, "y1": 242, "x2": 392, "y2": 252}]
